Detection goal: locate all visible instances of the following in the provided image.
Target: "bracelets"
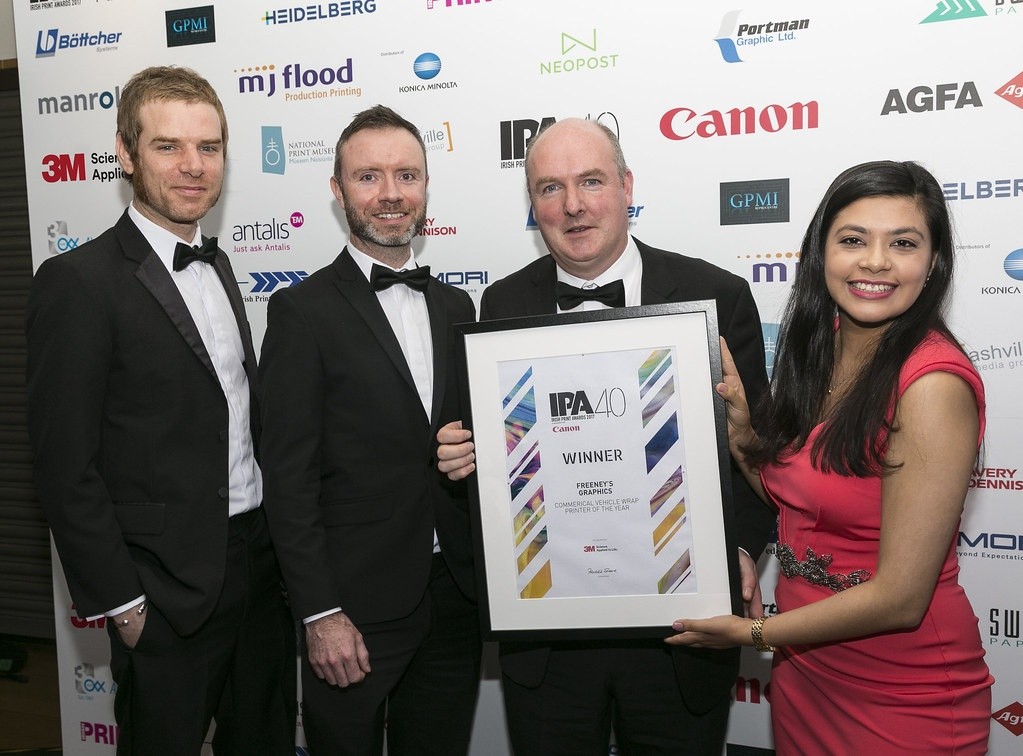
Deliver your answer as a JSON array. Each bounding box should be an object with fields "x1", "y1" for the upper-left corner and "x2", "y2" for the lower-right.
[
  {"x1": 752, "y1": 616, "x2": 778, "y2": 652},
  {"x1": 115, "y1": 601, "x2": 150, "y2": 628}
]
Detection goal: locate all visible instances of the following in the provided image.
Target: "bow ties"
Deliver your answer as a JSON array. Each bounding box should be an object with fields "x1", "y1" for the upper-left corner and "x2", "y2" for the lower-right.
[
  {"x1": 371, "y1": 263, "x2": 431, "y2": 292},
  {"x1": 172, "y1": 237, "x2": 218, "y2": 270},
  {"x1": 556, "y1": 280, "x2": 625, "y2": 311}
]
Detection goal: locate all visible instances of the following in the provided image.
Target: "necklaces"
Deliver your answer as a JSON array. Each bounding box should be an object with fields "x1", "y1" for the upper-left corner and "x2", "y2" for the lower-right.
[{"x1": 828, "y1": 376, "x2": 850, "y2": 395}]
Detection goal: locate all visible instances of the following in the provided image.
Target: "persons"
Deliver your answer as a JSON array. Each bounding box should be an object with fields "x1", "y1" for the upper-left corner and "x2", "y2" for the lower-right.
[
  {"x1": 666, "y1": 162, "x2": 996, "y2": 755},
  {"x1": 436, "y1": 118, "x2": 776, "y2": 755},
  {"x1": 25, "y1": 67, "x2": 295, "y2": 755},
  {"x1": 255, "y1": 105, "x2": 483, "y2": 756}
]
